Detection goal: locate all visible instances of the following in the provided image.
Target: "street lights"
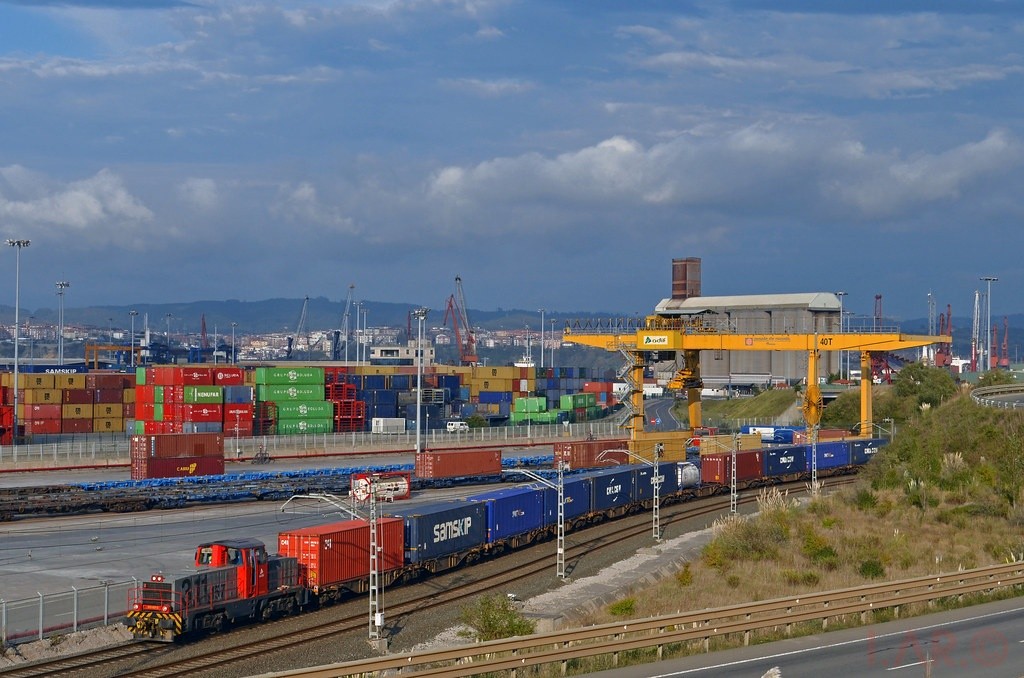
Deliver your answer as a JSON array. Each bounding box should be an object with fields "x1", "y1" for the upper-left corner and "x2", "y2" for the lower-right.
[
  {"x1": 412, "y1": 306, "x2": 432, "y2": 452},
  {"x1": 549, "y1": 319, "x2": 557, "y2": 367},
  {"x1": 231, "y1": 322, "x2": 239, "y2": 365},
  {"x1": 361, "y1": 309, "x2": 369, "y2": 364},
  {"x1": 3, "y1": 238, "x2": 32, "y2": 459},
  {"x1": 53, "y1": 280, "x2": 68, "y2": 364},
  {"x1": 165, "y1": 313, "x2": 172, "y2": 347},
  {"x1": 353, "y1": 303, "x2": 362, "y2": 365},
  {"x1": 980, "y1": 276, "x2": 998, "y2": 370},
  {"x1": 343, "y1": 313, "x2": 352, "y2": 365},
  {"x1": 129, "y1": 311, "x2": 139, "y2": 366},
  {"x1": 109, "y1": 318, "x2": 114, "y2": 355},
  {"x1": 834, "y1": 291, "x2": 848, "y2": 379},
  {"x1": 538, "y1": 308, "x2": 546, "y2": 367}
]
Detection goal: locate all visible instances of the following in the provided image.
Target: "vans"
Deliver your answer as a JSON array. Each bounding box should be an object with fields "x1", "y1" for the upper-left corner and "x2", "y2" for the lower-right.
[{"x1": 447, "y1": 422, "x2": 470, "y2": 433}]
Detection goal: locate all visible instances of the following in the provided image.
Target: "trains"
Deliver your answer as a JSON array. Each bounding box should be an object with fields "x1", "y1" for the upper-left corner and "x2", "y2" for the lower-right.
[{"x1": 122, "y1": 437, "x2": 889, "y2": 645}]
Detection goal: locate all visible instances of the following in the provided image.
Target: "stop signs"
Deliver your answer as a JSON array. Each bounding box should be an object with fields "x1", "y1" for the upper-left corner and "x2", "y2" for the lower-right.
[{"x1": 650, "y1": 419, "x2": 656, "y2": 426}]
